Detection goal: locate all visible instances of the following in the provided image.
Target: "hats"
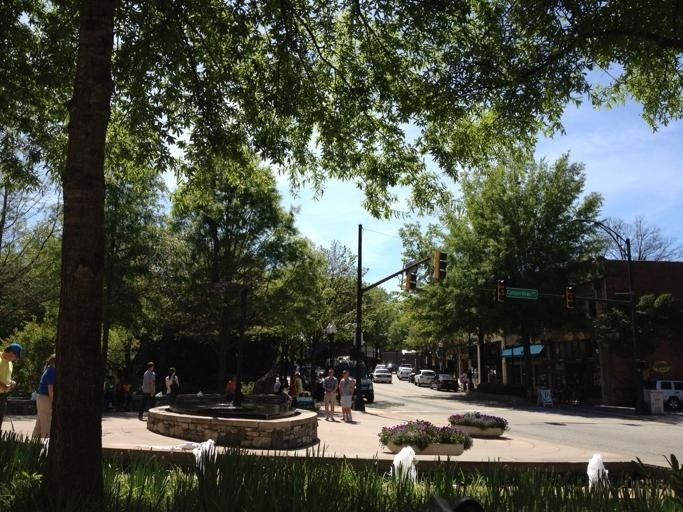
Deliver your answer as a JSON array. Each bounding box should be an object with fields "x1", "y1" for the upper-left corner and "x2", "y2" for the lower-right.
[{"x1": 9, "y1": 343, "x2": 22, "y2": 361}]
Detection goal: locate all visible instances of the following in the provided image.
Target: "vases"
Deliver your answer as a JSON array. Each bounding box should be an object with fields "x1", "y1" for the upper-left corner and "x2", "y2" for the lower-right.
[
  {"x1": 454, "y1": 425, "x2": 503, "y2": 438},
  {"x1": 387, "y1": 440, "x2": 463, "y2": 456}
]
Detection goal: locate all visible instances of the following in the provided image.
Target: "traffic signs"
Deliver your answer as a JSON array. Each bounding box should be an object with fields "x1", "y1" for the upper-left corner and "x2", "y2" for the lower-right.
[{"x1": 505, "y1": 287, "x2": 539, "y2": 300}]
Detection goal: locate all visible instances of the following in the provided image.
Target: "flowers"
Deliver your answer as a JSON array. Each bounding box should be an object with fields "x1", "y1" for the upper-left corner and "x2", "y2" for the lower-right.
[
  {"x1": 378, "y1": 420, "x2": 473, "y2": 450},
  {"x1": 447, "y1": 412, "x2": 508, "y2": 430}
]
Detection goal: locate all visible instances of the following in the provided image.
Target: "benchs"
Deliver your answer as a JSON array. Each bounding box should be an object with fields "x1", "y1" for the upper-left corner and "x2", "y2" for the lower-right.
[{"x1": 4, "y1": 392, "x2": 37, "y2": 411}]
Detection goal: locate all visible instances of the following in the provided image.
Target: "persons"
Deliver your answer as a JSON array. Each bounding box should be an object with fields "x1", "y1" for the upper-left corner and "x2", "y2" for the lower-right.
[
  {"x1": 165, "y1": 367, "x2": 180, "y2": 405},
  {"x1": 323, "y1": 369, "x2": 355, "y2": 422},
  {"x1": 105, "y1": 375, "x2": 133, "y2": 412},
  {"x1": 461, "y1": 369, "x2": 467, "y2": 390},
  {"x1": 0, "y1": 342, "x2": 22, "y2": 440},
  {"x1": 226, "y1": 375, "x2": 236, "y2": 404},
  {"x1": 137, "y1": 362, "x2": 156, "y2": 420},
  {"x1": 32, "y1": 353, "x2": 56, "y2": 438},
  {"x1": 273, "y1": 373, "x2": 303, "y2": 406}
]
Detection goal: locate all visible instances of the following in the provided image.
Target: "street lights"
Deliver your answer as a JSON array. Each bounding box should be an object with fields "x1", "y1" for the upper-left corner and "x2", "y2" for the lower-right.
[
  {"x1": 325, "y1": 321, "x2": 337, "y2": 369},
  {"x1": 343, "y1": 321, "x2": 358, "y2": 358},
  {"x1": 569, "y1": 219, "x2": 647, "y2": 414}
]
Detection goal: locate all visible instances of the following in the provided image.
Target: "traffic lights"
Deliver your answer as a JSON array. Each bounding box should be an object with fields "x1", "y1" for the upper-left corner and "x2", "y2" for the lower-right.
[
  {"x1": 433, "y1": 248, "x2": 448, "y2": 280},
  {"x1": 496, "y1": 279, "x2": 506, "y2": 303},
  {"x1": 406, "y1": 267, "x2": 419, "y2": 290},
  {"x1": 565, "y1": 285, "x2": 577, "y2": 309}
]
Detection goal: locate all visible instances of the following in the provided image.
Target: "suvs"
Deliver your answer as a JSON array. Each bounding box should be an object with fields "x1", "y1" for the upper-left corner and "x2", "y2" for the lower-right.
[
  {"x1": 413, "y1": 369, "x2": 436, "y2": 387},
  {"x1": 643, "y1": 379, "x2": 683, "y2": 412},
  {"x1": 337, "y1": 359, "x2": 375, "y2": 404}
]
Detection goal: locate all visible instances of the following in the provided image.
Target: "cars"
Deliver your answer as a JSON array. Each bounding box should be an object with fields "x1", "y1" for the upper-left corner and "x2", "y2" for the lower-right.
[
  {"x1": 371, "y1": 363, "x2": 416, "y2": 384},
  {"x1": 429, "y1": 374, "x2": 459, "y2": 392}
]
